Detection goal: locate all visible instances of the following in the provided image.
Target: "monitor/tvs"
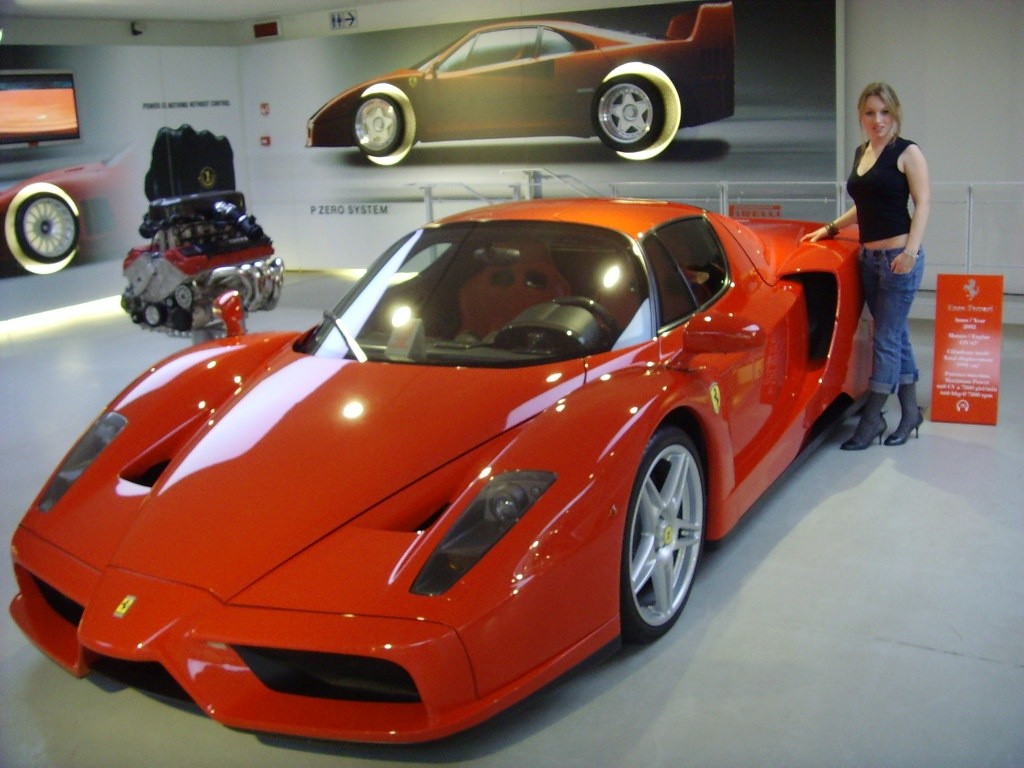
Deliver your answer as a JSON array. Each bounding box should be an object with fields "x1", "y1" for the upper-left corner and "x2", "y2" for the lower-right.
[{"x1": 0, "y1": 73, "x2": 80, "y2": 145}]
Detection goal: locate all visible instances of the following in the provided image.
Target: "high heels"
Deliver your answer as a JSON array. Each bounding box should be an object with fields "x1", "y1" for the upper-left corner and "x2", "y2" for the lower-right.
[
  {"x1": 841, "y1": 417, "x2": 887, "y2": 450},
  {"x1": 884, "y1": 406, "x2": 923, "y2": 445}
]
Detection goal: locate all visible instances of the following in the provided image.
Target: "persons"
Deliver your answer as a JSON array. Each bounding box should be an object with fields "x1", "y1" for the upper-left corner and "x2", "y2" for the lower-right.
[{"x1": 800, "y1": 82, "x2": 931, "y2": 451}]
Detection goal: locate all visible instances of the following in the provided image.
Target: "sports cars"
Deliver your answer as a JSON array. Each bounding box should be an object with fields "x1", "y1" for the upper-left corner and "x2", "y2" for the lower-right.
[
  {"x1": 306, "y1": 2, "x2": 739, "y2": 166},
  {"x1": 8, "y1": 196, "x2": 863, "y2": 749},
  {"x1": 0, "y1": 140, "x2": 162, "y2": 276}
]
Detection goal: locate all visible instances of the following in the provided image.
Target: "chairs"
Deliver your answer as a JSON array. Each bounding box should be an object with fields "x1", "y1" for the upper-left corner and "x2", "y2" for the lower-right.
[{"x1": 457, "y1": 240, "x2": 707, "y2": 341}]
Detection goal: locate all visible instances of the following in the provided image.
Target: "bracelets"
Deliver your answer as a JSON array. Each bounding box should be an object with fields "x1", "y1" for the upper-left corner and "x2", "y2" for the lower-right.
[
  {"x1": 824, "y1": 221, "x2": 840, "y2": 237},
  {"x1": 903, "y1": 250, "x2": 920, "y2": 259}
]
